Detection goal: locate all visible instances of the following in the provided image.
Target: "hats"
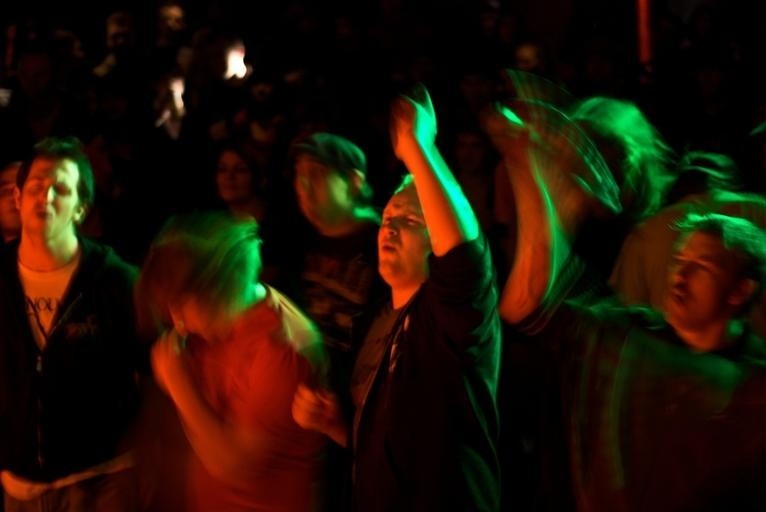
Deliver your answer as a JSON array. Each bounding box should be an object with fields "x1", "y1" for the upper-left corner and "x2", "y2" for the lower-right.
[{"x1": 292, "y1": 132, "x2": 374, "y2": 198}]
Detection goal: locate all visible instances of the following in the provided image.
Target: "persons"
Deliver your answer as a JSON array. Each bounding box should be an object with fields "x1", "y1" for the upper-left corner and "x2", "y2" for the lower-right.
[{"x1": 0, "y1": 6, "x2": 766, "y2": 512}]
